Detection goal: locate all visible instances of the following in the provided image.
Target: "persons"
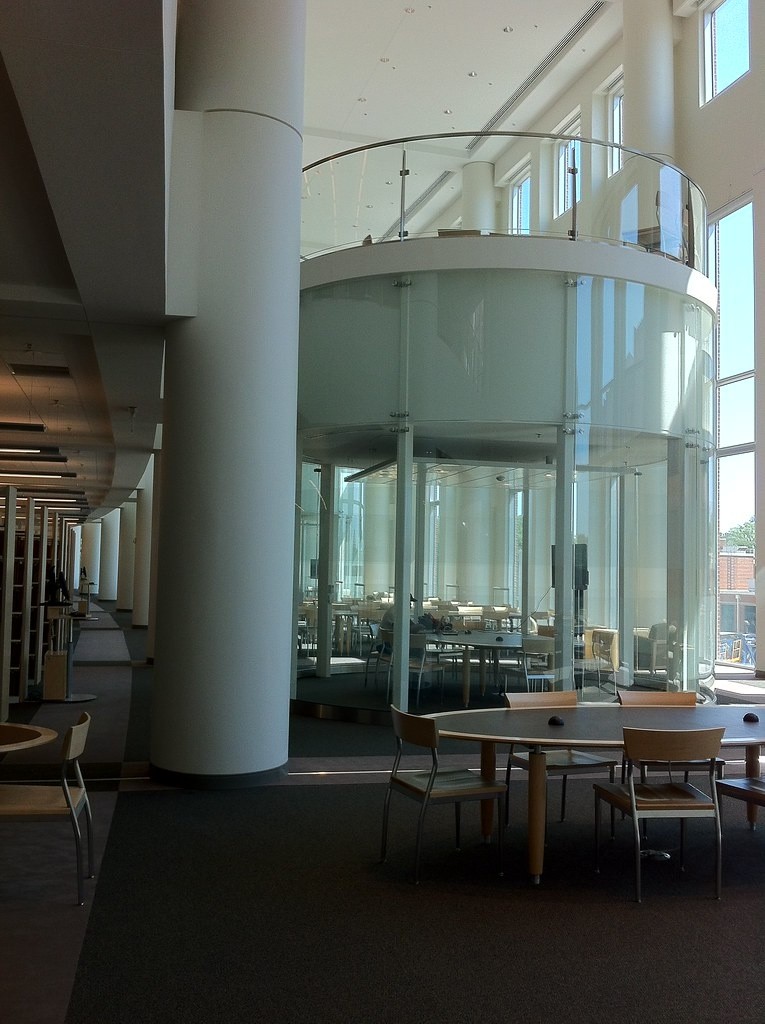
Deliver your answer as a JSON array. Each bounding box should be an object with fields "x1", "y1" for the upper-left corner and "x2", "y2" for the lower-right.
[{"x1": 375, "y1": 593, "x2": 431, "y2": 689}]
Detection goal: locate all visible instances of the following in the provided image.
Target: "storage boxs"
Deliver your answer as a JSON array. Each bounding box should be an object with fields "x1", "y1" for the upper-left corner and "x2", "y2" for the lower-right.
[
  {"x1": 41, "y1": 651, "x2": 68, "y2": 702},
  {"x1": 78, "y1": 601, "x2": 87, "y2": 614}
]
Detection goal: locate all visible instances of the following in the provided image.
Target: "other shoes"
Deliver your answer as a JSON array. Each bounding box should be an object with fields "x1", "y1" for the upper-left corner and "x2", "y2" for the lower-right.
[{"x1": 411, "y1": 681, "x2": 432, "y2": 690}]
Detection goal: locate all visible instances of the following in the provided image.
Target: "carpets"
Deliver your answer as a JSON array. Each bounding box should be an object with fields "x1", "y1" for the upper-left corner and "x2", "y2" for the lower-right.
[{"x1": 62, "y1": 772, "x2": 765, "y2": 1024}]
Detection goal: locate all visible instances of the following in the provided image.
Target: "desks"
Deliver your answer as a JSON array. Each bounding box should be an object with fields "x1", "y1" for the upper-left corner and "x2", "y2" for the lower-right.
[
  {"x1": 420, "y1": 630, "x2": 548, "y2": 707},
  {"x1": 444, "y1": 609, "x2": 482, "y2": 625},
  {"x1": 417, "y1": 704, "x2": 765, "y2": 884},
  {"x1": 60, "y1": 615, "x2": 99, "y2": 701},
  {"x1": 79, "y1": 583, "x2": 99, "y2": 620},
  {"x1": 0, "y1": 722, "x2": 59, "y2": 753},
  {"x1": 334, "y1": 610, "x2": 358, "y2": 656}
]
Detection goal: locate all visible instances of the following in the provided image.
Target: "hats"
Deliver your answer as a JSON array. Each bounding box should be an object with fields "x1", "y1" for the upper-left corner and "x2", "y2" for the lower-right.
[{"x1": 410, "y1": 593, "x2": 418, "y2": 602}]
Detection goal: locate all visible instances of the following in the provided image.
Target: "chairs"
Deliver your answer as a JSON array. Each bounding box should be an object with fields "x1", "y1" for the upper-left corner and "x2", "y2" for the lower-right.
[
  {"x1": 0, "y1": 711, "x2": 94, "y2": 907},
  {"x1": 296, "y1": 585, "x2": 765, "y2": 904}
]
